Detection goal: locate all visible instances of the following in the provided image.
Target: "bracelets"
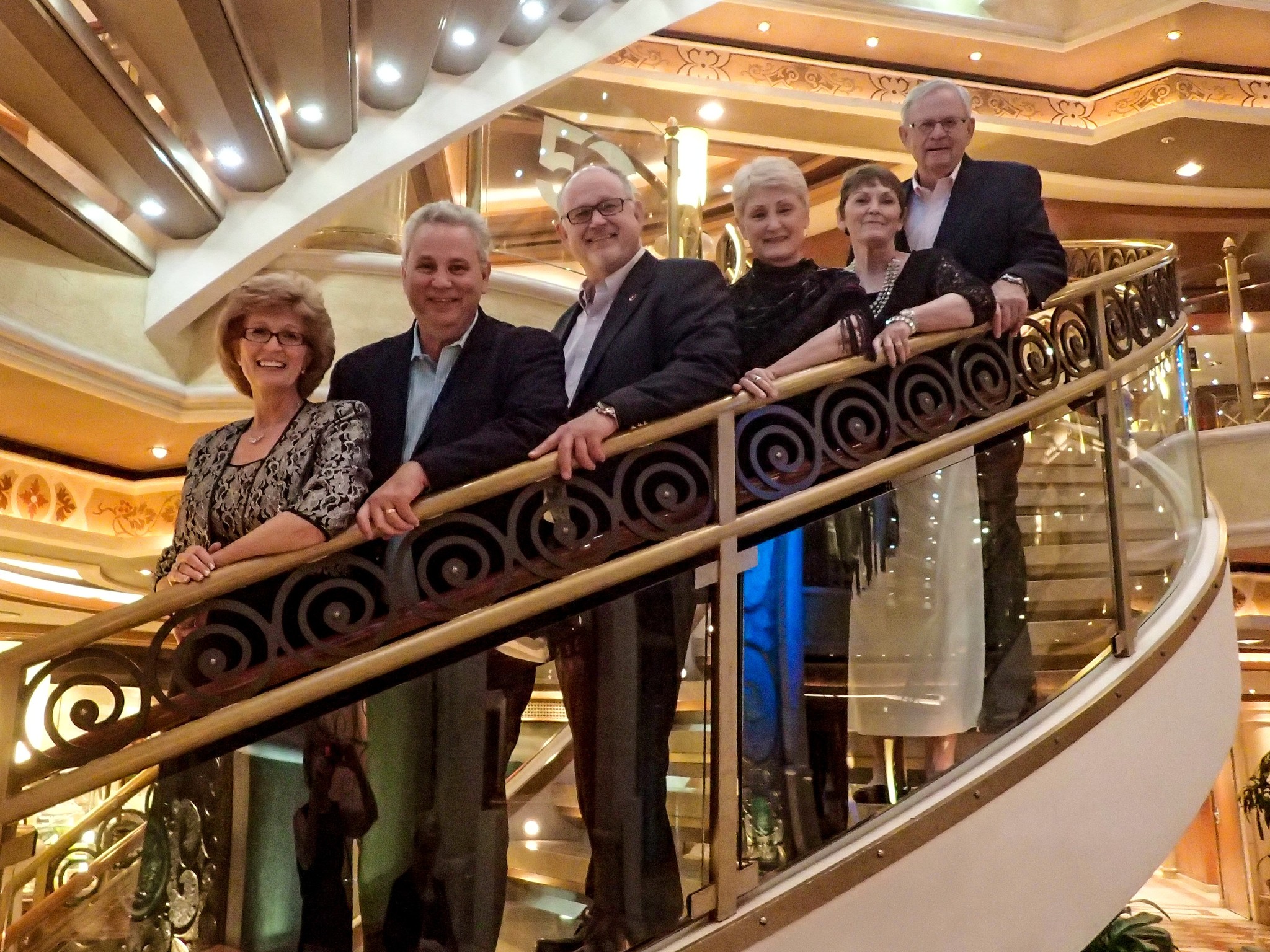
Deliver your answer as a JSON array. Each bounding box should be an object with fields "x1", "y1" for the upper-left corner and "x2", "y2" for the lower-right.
[{"x1": 884, "y1": 315, "x2": 917, "y2": 336}]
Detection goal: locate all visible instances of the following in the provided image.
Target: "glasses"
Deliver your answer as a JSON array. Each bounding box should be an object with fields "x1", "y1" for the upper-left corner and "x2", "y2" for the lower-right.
[
  {"x1": 561, "y1": 198, "x2": 632, "y2": 224},
  {"x1": 906, "y1": 117, "x2": 967, "y2": 134},
  {"x1": 237, "y1": 328, "x2": 311, "y2": 347}
]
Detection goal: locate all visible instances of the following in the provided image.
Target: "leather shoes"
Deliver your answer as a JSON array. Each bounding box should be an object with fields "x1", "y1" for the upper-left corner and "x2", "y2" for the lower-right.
[
  {"x1": 573, "y1": 911, "x2": 634, "y2": 952},
  {"x1": 536, "y1": 906, "x2": 603, "y2": 951}
]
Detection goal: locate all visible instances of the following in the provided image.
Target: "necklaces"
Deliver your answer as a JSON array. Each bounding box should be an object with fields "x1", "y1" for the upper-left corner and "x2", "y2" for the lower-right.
[
  {"x1": 842, "y1": 257, "x2": 901, "y2": 320},
  {"x1": 247, "y1": 399, "x2": 302, "y2": 443}
]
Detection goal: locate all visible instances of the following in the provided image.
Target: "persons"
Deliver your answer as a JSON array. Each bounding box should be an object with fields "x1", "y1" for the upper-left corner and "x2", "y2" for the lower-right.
[
  {"x1": 293, "y1": 725, "x2": 379, "y2": 952},
  {"x1": 836, "y1": 165, "x2": 995, "y2": 805},
  {"x1": 893, "y1": 79, "x2": 1069, "y2": 735},
  {"x1": 383, "y1": 821, "x2": 460, "y2": 952},
  {"x1": 323, "y1": 196, "x2": 570, "y2": 952},
  {"x1": 726, "y1": 152, "x2": 876, "y2": 870},
  {"x1": 125, "y1": 267, "x2": 369, "y2": 952},
  {"x1": 527, "y1": 160, "x2": 744, "y2": 952}
]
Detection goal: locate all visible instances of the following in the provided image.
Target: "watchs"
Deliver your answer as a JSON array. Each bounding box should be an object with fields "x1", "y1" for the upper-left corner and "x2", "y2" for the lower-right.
[
  {"x1": 994, "y1": 273, "x2": 1030, "y2": 297},
  {"x1": 896, "y1": 308, "x2": 920, "y2": 333},
  {"x1": 595, "y1": 400, "x2": 620, "y2": 430}
]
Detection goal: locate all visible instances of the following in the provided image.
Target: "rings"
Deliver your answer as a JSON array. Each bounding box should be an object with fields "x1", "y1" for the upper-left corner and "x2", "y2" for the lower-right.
[
  {"x1": 752, "y1": 375, "x2": 761, "y2": 382},
  {"x1": 384, "y1": 509, "x2": 396, "y2": 514},
  {"x1": 175, "y1": 561, "x2": 183, "y2": 572}
]
